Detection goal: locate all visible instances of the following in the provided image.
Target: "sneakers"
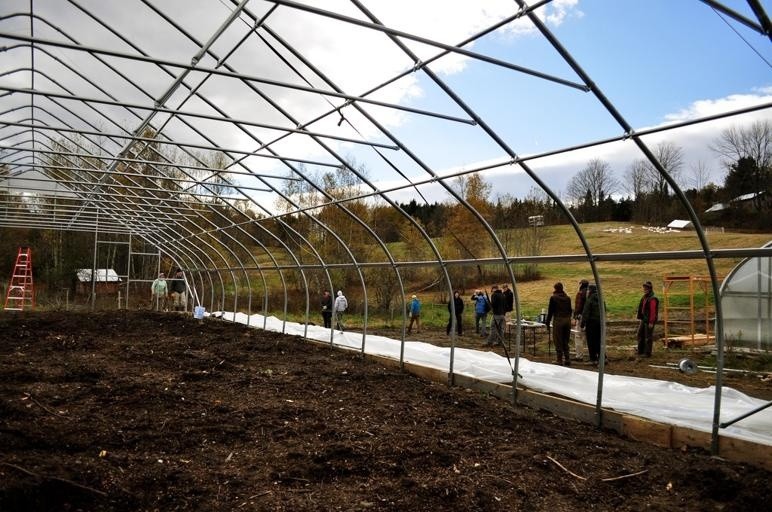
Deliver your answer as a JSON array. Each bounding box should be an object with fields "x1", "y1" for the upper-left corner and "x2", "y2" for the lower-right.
[
  {"x1": 640, "y1": 354, "x2": 648, "y2": 358},
  {"x1": 552, "y1": 360, "x2": 563, "y2": 364},
  {"x1": 583, "y1": 359, "x2": 598, "y2": 366}
]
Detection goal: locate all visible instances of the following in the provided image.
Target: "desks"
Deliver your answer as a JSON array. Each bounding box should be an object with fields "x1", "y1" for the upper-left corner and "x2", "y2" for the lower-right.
[{"x1": 505, "y1": 320, "x2": 554, "y2": 358}]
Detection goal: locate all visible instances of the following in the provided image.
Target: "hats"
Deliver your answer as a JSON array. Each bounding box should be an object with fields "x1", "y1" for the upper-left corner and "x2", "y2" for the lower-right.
[
  {"x1": 412, "y1": 295, "x2": 416, "y2": 298},
  {"x1": 338, "y1": 291, "x2": 342, "y2": 295}
]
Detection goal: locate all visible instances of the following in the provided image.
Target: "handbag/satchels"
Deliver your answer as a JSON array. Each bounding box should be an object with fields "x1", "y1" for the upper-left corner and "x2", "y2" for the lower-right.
[{"x1": 483, "y1": 296, "x2": 492, "y2": 313}]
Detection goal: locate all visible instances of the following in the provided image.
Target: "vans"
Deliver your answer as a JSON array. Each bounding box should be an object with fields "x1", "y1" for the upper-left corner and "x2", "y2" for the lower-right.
[{"x1": 528, "y1": 215, "x2": 544, "y2": 226}]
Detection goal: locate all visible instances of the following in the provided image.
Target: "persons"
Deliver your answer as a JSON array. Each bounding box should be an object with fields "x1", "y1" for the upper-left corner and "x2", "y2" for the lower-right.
[
  {"x1": 171, "y1": 268, "x2": 187, "y2": 311},
  {"x1": 636, "y1": 281, "x2": 659, "y2": 357},
  {"x1": 335, "y1": 291, "x2": 348, "y2": 331},
  {"x1": 470, "y1": 285, "x2": 514, "y2": 349},
  {"x1": 320, "y1": 290, "x2": 333, "y2": 328},
  {"x1": 546, "y1": 280, "x2": 609, "y2": 367},
  {"x1": 407, "y1": 295, "x2": 421, "y2": 333},
  {"x1": 151, "y1": 272, "x2": 168, "y2": 310},
  {"x1": 446, "y1": 291, "x2": 464, "y2": 336}
]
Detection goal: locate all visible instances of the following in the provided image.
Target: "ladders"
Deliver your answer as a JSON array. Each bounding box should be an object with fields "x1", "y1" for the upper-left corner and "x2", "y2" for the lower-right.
[{"x1": 3, "y1": 247, "x2": 36, "y2": 311}]
